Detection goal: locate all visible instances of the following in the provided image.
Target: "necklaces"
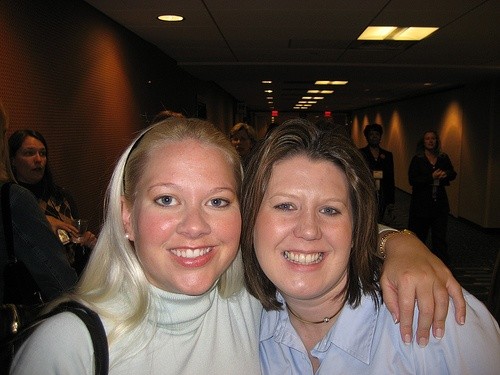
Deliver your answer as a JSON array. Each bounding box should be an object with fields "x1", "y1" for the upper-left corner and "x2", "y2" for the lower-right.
[{"x1": 286, "y1": 300, "x2": 345, "y2": 323}]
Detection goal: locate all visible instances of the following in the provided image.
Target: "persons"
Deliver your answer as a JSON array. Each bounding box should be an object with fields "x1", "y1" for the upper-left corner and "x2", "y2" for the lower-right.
[
  {"x1": 241, "y1": 119, "x2": 500, "y2": 374},
  {"x1": 0, "y1": 110, "x2": 456, "y2": 317},
  {"x1": 8, "y1": 118, "x2": 466, "y2": 375}
]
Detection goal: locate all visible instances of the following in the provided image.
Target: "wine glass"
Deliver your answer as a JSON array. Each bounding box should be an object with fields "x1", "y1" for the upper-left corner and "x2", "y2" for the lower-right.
[{"x1": 73, "y1": 217, "x2": 88, "y2": 253}]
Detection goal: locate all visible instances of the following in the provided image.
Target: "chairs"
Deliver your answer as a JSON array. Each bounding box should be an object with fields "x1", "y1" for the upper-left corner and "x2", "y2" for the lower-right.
[{"x1": 449, "y1": 241, "x2": 500, "y2": 309}]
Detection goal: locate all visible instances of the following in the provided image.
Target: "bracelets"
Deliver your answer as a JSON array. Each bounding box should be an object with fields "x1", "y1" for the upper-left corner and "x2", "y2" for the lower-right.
[{"x1": 379, "y1": 229, "x2": 413, "y2": 258}]
